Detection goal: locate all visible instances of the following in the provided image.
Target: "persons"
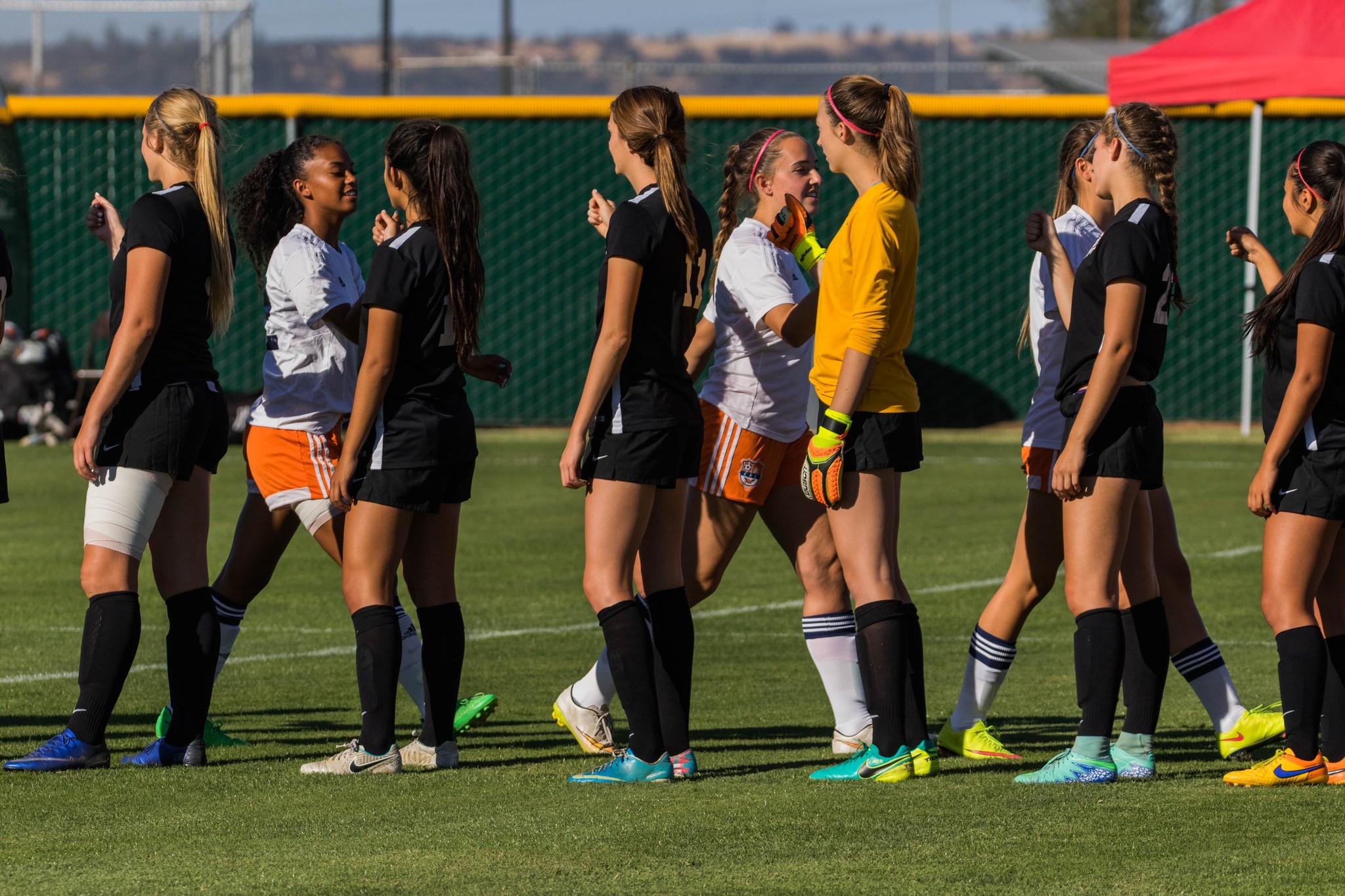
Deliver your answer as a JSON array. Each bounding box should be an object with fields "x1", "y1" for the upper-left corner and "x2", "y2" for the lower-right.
[
  {"x1": 935, "y1": 122, "x2": 1283, "y2": 761},
  {"x1": 552, "y1": 129, "x2": 871, "y2": 752},
  {"x1": 152, "y1": 135, "x2": 499, "y2": 748},
  {"x1": 1010, "y1": 101, "x2": 1187, "y2": 784},
  {"x1": 1220, "y1": 140, "x2": 1345, "y2": 787},
  {"x1": 769, "y1": 74, "x2": 939, "y2": 781},
  {"x1": 0, "y1": 88, "x2": 237, "y2": 771},
  {"x1": 300, "y1": 119, "x2": 486, "y2": 774},
  {"x1": 559, "y1": 87, "x2": 716, "y2": 784}
]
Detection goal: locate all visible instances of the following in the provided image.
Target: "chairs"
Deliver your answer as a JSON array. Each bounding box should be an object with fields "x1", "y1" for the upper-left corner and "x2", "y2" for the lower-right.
[{"x1": 69, "y1": 309, "x2": 112, "y2": 434}]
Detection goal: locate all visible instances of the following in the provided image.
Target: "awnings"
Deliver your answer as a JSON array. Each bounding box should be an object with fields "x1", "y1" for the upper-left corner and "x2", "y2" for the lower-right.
[{"x1": 1107, "y1": 17, "x2": 1342, "y2": 434}]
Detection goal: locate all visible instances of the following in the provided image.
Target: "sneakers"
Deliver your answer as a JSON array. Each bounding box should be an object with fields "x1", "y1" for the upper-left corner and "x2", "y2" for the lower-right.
[
  {"x1": 936, "y1": 718, "x2": 1021, "y2": 761},
  {"x1": 1, "y1": 729, "x2": 110, "y2": 772},
  {"x1": 156, "y1": 707, "x2": 253, "y2": 749},
  {"x1": 551, "y1": 682, "x2": 622, "y2": 755},
  {"x1": 831, "y1": 725, "x2": 874, "y2": 755},
  {"x1": 566, "y1": 749, "x2": 700, "y2": 783},
  {"x1": 1213, "y1": 708, "x2": 1286, "y2": 761},
  {"x1": 299, "y1": 738, "x2": 402, "y2": 774},
  {"x1": 119, "y1": 736, "x2": 207, "y2": 768},
  {"x1": 808, "y1": 738, "x2": 939, "y2": 783},
  {"x1": 1322, "y1": 756, "x2": 1345, "y2": 786},
  {"x1": 419, "y1": 692, "x2": 499, "y2": 741},
  {"x1": 1222, "y1": 748, "x2": 1330, "y2": 788},
  {"x1": 398, "y1": 740, "x2": 459, "y2": 771},
  {"x1": 1014, "y1": 748, "x2": 1118, "y2": 786},
  {"x1": 1111, "y1": 745, "x2": 1156, "y2": 780}
]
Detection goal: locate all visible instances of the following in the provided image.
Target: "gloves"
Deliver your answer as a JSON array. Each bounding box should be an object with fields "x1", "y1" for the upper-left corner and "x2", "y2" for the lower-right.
[
  {"x1": 799, "y1": 408, "x2": 852, "y2": 508},
  {"x1": 765, "y1": 194, "x2": 827, "y2": 273}
]
